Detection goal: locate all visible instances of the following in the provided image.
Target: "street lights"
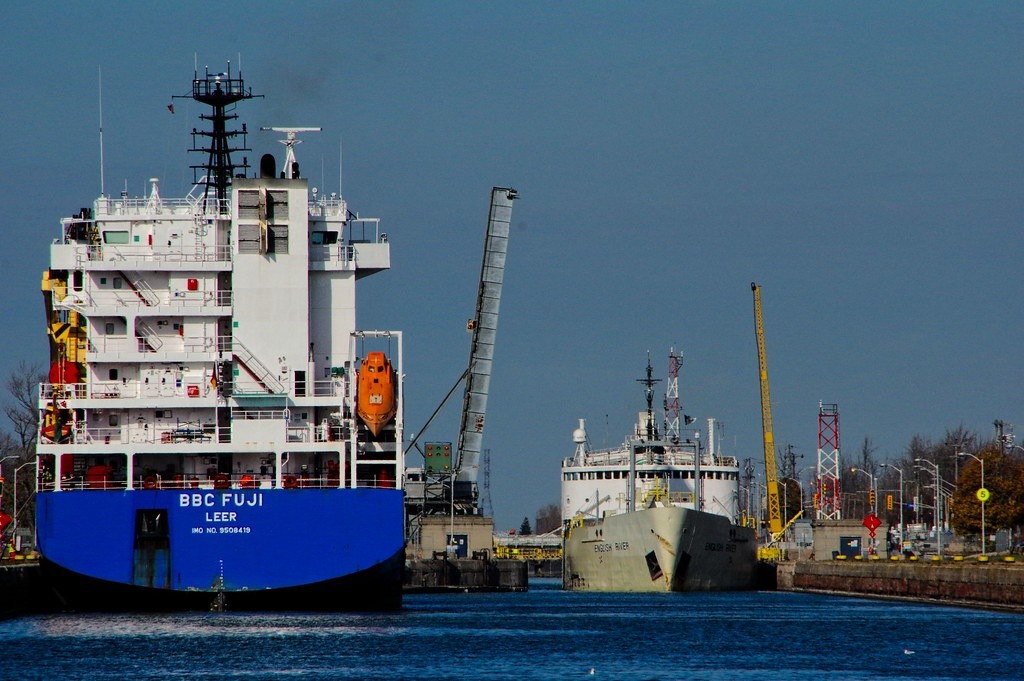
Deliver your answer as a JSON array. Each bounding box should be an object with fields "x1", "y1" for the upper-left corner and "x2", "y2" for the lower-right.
[
  {"x1": 913, "y1": 465, "x2": 944, "y2": 532},
  {"x1": 13, "y1": 462, "x2": 36, "y2": 545},
  {"x1": 879, "y1": 463, "x2": 905, "y2": 561},
  {"x1": 769, "y1": 479, "x2": 787, "y2": 543},
  {"x1": 738, "y1": 475, "x2": 768, "y2": 524},
  {"x1": 789, "y1": 477, "x2": 803, "y2": 520},
  {"x1": 913, "y1": 458, "x2": 942, "y2": 561},
  {"x1": 796, "y1": 466, "x2": 814, "y2": 482},
  {"x1": 957, "y1": 452, "x2": 989, "y2": 563},
  {"x1": 849, "y1": 466, "x2": 873, "y2": 515}
]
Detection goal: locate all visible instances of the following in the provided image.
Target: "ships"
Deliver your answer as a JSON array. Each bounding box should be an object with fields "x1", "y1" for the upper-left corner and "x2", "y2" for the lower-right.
[
  {"x1": 30, "y1": 51, "x2": 410, "y2": 613},
  {"x1": 560, "y1": 345, "x2": 759, "y2": 592}
]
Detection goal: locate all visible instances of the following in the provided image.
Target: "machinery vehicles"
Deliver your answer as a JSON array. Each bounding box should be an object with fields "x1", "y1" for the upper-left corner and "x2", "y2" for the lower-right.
[{"x1": 405, "y1": 184, "x2": 523, "y2": 518}]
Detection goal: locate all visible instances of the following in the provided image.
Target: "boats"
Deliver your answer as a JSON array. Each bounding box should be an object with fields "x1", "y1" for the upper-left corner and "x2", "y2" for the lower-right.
[{"x1": 356, "y1": 351, "x2": 397, "y2": 438}]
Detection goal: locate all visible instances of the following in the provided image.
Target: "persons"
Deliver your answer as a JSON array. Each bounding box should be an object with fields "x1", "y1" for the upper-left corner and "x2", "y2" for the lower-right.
[
  {"x1": 7, "y1": 539, "x2": 16, "y2": 564},
  {"x1": 663, "y1": 434, "x2": 681, "y2": 452},
  {"x1": 888, "y1": 525, "x2": 925, "y2": 557}
]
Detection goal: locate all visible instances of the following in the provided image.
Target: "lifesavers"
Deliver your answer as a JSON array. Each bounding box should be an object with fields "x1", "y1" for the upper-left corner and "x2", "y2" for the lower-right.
[
  {"x1": 241, "y1": 475, "x2": 254, "y2": 489},
  {"x1": 144, "y1": 476, "x2": 157, "y2": 489},
  {"x1": 310, "y1": 223, "x2": 319, "y2": 233},
  {"x1": 313, "y1": 232, "x2": 324, "y2": 243},
  {"x1": 213, "y1": 475, "x2": 229, "y2": 489},
  {"x1": 335, "y1": 473, "x2": 347, "y2": 485},
  {"x1": 284, "y1": 475, "x2": 298, "y2": 488}
]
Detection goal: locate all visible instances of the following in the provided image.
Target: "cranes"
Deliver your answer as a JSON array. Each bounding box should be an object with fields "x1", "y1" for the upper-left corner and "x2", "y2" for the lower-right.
[{"x1": 751, "y1": 281, "x2": 785, "y2": 545}]
